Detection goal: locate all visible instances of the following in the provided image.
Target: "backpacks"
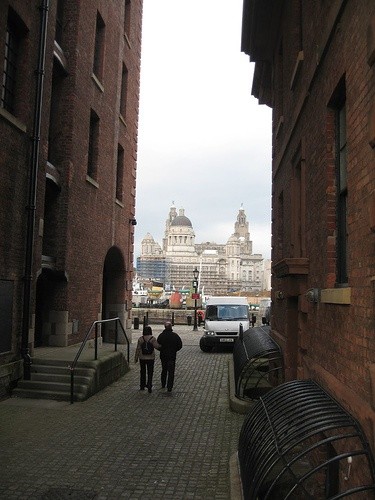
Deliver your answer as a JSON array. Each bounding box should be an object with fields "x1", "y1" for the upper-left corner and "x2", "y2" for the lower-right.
[
  {"x1": 164, "y1": 332, "x2": 182, "y2": 352},
  {"x1": 141, "y1": 336, "x2": 154, "y2": 355}
]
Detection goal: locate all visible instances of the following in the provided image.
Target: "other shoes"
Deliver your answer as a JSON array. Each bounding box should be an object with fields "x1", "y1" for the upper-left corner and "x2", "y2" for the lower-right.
[
  {"x1": 140, "y1": 387, "x2": 144, "y2": 390},
  {"x1": 162, "y1": 385, "x2": 165, "y2": 388},
  {"x1": 168, "y1": 388, "x2": 172, "y2": 392},
  {"x1": 148, "y1": 389, "x2": 152, "y2": 393}
]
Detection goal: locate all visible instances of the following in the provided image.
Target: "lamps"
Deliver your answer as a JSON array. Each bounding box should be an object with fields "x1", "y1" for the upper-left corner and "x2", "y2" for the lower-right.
[{"x1": 129, "y1": 219, "x2": 136, "y2": 225}]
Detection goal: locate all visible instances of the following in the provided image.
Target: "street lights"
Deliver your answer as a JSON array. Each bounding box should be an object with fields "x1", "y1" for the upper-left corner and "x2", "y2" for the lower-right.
[{"x1": 192, "y1": 267, "x2": 200, "y2": 331}]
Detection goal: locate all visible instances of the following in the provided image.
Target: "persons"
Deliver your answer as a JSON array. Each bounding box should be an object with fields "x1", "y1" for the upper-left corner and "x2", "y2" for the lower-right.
[
  {"x1": 187, "y1": 313, "x2": 192, "y2": 326},
  {"x1": 134, "y1": 327, "x2": 158, "y2": 393},
  {"x1": 156, "y1": 322, "x2": 182, "y2": 394}
]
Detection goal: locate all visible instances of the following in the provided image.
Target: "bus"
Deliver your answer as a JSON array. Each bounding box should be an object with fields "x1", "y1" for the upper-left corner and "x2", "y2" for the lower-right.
[{"x1": 259, "y1": 297, "x2": 272, "y2": 324}]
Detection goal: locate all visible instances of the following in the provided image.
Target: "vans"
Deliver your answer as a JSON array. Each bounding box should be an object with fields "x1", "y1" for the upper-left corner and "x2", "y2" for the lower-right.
[{"x1": 199, "y1": 296, "x2": 257, "y2": 353}]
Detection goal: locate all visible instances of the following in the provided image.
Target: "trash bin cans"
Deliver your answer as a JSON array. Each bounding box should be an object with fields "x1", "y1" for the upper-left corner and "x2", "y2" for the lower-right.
[
  {"x1": 134, "y1": 318, "x2": 139, "y2": 329},
  {"x1": 187, "y1": 316, "x2": 192, "y2": 326}
]
